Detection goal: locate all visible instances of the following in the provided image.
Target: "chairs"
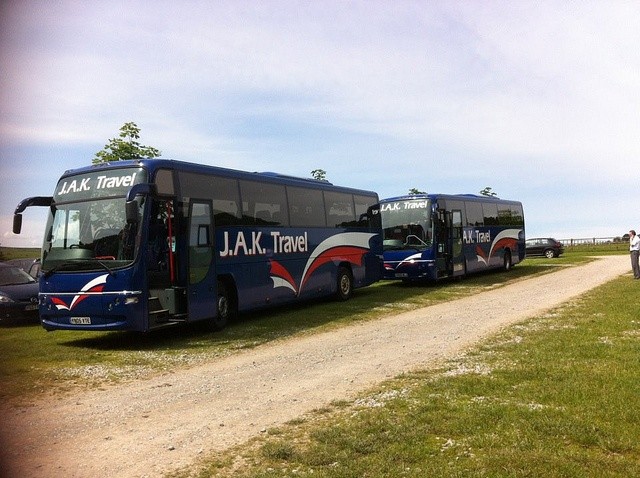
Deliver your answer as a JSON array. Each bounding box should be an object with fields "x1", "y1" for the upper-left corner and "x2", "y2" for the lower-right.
[
  {"x1": 256, "y1": 210, "x2": 271, "y2": 225},
  {"x1": 272, "y1": 212, "x2": 282, "y2": 222}
]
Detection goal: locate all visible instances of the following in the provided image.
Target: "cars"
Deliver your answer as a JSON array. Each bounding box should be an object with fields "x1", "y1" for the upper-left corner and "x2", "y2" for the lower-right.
[
  {"x1": 0, "y1": 263, "x2": 39, "y2": 318},
  {"x1": 6, "y1": 258, "x2": 40, "y2": 279}
]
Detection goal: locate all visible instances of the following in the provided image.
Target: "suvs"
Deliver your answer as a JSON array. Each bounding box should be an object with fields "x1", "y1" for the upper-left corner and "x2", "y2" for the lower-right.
[{"x1": 525, "y1": 238, "x2": 564, "y2": 258}]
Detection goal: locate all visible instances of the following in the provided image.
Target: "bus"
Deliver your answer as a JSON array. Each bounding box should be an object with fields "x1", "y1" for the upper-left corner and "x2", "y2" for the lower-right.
[
  {"x1": 379, "y1": 193, "x2": 526, "y2": 280},
  {"x1": 12, "y1": 159, "x2": 383, "y2": 331}
]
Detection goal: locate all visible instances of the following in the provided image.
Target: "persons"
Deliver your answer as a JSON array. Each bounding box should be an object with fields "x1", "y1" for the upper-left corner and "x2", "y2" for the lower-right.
[{"x1": 629, "y1": 230, "x2": 640, "y2": 279}]
